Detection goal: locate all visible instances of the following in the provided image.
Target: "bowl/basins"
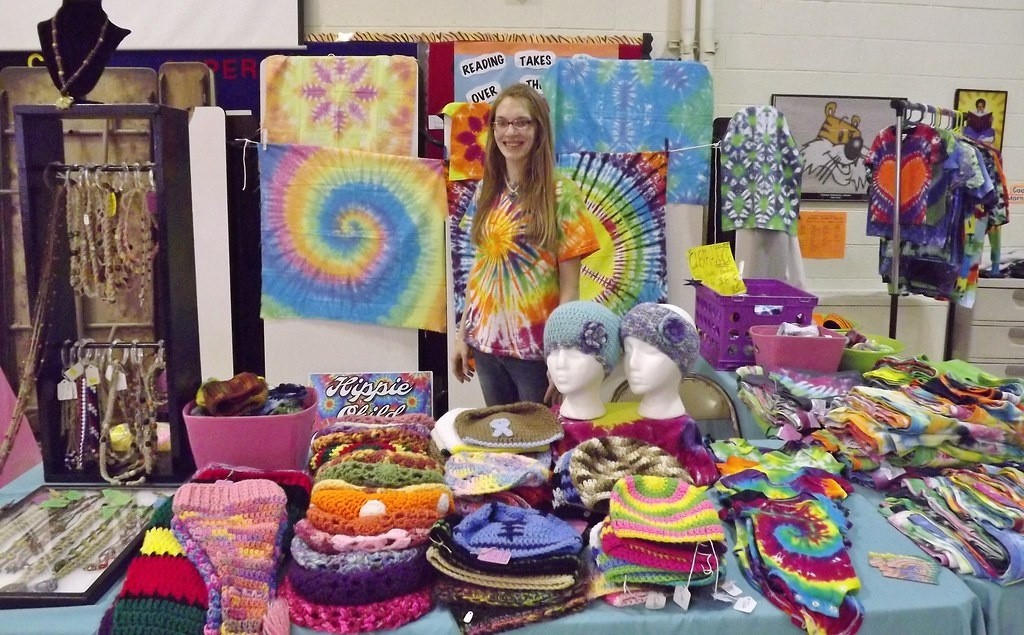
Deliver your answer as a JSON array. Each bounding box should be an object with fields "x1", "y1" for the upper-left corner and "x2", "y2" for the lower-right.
[
  {"x1": 750, "y1": 324, "x2": 847, "y2": 375},
  {"x1": 826, "y1": 327, "x2": 905, "y2": 371},
  {"x1": 182, "y1": 386, "x2": 318, "y2": 473}
]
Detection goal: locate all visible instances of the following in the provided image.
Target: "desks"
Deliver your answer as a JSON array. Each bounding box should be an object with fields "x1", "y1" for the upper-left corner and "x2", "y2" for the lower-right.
[{"x1": 0, "y1": 444, "x2": 1023, "y2": 635}]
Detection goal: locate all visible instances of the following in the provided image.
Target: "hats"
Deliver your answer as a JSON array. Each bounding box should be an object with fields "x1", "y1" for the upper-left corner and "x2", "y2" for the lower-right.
[
  {"x1": 98, "y1": 374, "x2": 726, "y2": 635},
  {"x1": 542, "y1": 300, "x2": 622, "y2": 380},
  {"x1": 620, "y1": 302, "x2": 700, "y2": 378}
]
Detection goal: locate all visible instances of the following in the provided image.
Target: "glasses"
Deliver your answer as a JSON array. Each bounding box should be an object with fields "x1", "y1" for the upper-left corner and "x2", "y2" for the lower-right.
[{"x1": 492, "y1": 117, "x2": 538, "y2": 130}]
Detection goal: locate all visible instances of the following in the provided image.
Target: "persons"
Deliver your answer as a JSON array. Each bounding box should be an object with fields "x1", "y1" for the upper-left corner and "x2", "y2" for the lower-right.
[
  {"x1": 618, "y1": 302, "x2": 702, "y2": 420},
  {"x1": 452, "y1": 83, "x2": 600, "y2": 410},
  {"x1": 543, "y1": 300, "x2": 625, "y2": 420}
]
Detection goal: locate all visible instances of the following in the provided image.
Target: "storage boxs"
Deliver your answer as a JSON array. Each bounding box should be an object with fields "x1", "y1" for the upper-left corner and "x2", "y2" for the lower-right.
[
  {"x1": 749, "y1": 325, "x2": 844, "y2": 372},
  {"x1": 693, "y1": 279, "x2": 819, "y2": 370},
  {"x1": 184, "y1": 387, "x2": 318, "y2": 472}
]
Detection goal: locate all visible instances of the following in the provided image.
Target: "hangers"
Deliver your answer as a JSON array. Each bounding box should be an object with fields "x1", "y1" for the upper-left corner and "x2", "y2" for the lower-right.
[{"x1": 895, "y1": 103, "x2": 965, "y2": 135}]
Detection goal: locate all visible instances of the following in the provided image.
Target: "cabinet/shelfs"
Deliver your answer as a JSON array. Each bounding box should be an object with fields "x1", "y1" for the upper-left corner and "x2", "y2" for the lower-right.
[{"x1": 951, "y1": 278, "x2": 1024, "y2": 380}]
